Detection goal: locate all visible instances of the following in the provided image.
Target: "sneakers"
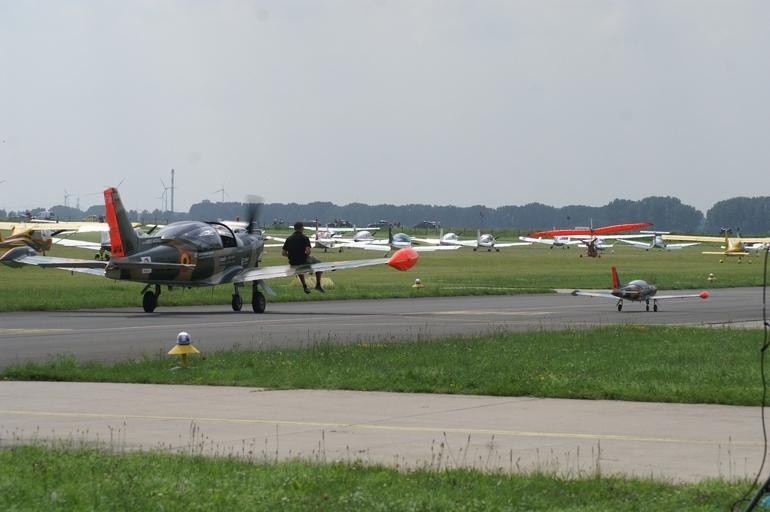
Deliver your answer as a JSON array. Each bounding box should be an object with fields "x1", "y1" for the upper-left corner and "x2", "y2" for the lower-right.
[
  {"x1": 304, "y1": 289, "x2": 310, "y2": 292},
  {"x1": 316, "y1": 286, "x2": 323, "y2": 290}
]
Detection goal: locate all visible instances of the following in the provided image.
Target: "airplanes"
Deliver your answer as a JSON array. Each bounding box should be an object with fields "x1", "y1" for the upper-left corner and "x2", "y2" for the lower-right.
[
  {"x1": 554, "y1": 267, "x2": 710, "y2": 312},
  {"x1": 440, "y1": 229, "x2": 532, "y2": 253},
  {"x1": 520, "y1": 224, "x2": 766, "y2": 259}
]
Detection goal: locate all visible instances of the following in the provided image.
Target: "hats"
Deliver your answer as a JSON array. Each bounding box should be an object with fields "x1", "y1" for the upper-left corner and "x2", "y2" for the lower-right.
[{"x1": 294, "y1": 223, "x2": 303, "y2": 230}]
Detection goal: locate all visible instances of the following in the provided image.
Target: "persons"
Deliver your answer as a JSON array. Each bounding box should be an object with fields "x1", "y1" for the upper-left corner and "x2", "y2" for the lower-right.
[{"x1": 281, "y1": 222, "x2": 326, "y2": 294}]
